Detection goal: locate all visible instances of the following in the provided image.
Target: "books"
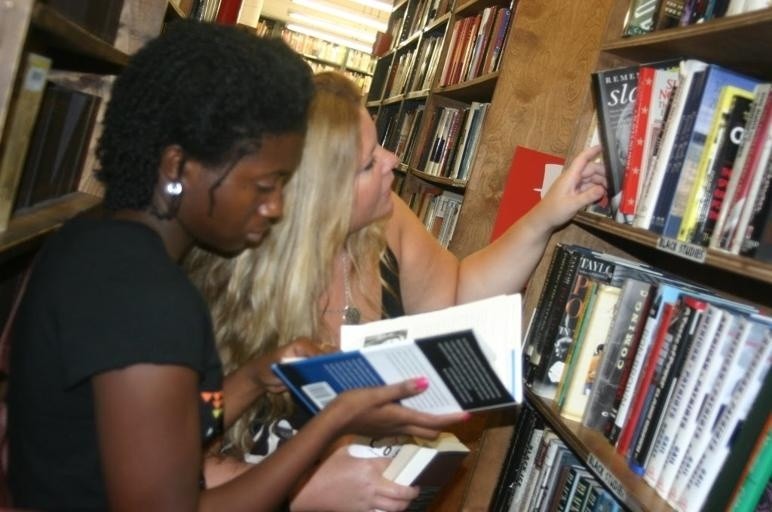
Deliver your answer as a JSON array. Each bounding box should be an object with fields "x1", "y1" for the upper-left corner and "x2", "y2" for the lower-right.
[
  {"x1": 487, "y1": 243, "x2": 771, "y2": 512},
  {"x1": 592, "y1": 57, "x2": 771, "y2": 262},
  {"x1": 376, "y1": 433, "x2": 471, "y2": 512},
  {"x1": 369, "y1": 0, "x2": 516, "y2": 316},
  {"x1": 255, "y1": 20, "x2": 380, "y2": 94},
  {"x1": 622, "y1": 0, "x2": 772, "y2": 37},
  {"x1": 1, "y1": 0, "x2": 221, "y2": 376},
  {"x1": 271, "y1": 292, "x2": 524, "y2": 419}
]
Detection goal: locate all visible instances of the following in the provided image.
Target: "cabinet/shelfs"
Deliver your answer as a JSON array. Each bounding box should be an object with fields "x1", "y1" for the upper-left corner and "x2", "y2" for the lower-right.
[{"x1": 0, "y1": 1, "x2": 772, "y2": 512}]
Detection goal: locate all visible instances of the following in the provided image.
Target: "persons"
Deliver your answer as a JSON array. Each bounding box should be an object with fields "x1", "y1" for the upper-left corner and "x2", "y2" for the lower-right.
[
  {"x1": 1, "y1": 21, "x2": 475, "y2": 511},
  {"x1": 185, "y1": 71, "x2": 606, "y2": 510}
]
